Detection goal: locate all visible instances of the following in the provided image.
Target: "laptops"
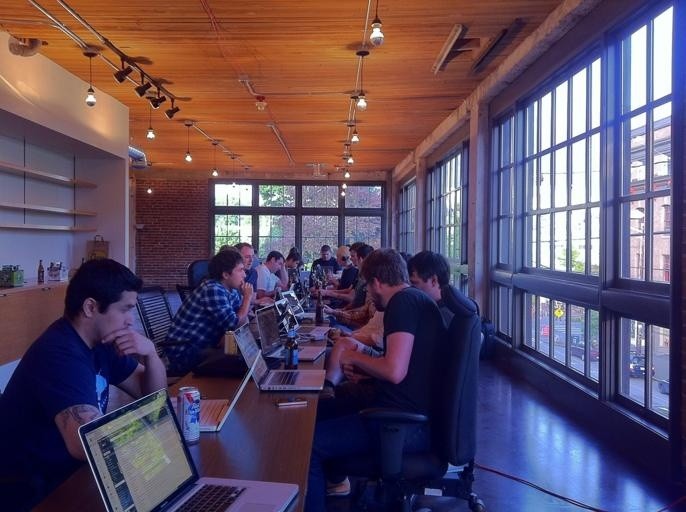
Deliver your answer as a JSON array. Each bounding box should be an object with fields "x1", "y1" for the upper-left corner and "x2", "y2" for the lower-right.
[
  {"x1": 78, "y1": 389, "x2": 299, "y2": 512},
  {"x1": 274, "y1": 298, "x2": 332, "y2": 337},
  {"x1": 281, "y1": 265, "x2": 333, "y2": 321},
  {"x1": 169, "y1": 350, "x2": 262, "y2": 432},
  {"x1": 255, "y1": 303, "x2": 326, "y2": 362},
  {"x1": 230, "y1": 323, "x2": 327, "y2": 391}
]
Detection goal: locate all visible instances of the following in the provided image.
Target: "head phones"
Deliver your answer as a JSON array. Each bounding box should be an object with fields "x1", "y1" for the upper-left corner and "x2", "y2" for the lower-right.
[{"x1": 341, "y1": 243, "x2": 352, "y2": 261}]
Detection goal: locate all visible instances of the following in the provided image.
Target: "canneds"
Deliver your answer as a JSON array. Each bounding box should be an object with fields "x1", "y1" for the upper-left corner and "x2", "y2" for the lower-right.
[{"x1": 177, "y1": 386, "x2": 201, "y2": 445}]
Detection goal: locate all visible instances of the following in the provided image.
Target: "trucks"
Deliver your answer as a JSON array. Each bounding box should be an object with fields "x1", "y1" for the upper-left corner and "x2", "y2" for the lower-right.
[{"x1": 535, "y1": 315, "x2": 581, "y2": 346}]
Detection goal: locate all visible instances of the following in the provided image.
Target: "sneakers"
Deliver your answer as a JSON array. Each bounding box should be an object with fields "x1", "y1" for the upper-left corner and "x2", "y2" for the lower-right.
[{"x1": 327, "y1": 476, "x2": 351, "y2": 496}]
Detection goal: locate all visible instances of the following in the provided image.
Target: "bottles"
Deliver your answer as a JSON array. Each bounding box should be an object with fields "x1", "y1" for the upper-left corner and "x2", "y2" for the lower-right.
[
  {"x1": 322, "y1": 280, "x2": 327, "y2": 289},
  {"x1": 315, "y1": 291, "x2": 323, "y2": 326},
  {"x1": 37, "y1": 259, "x2": 44, "y2": 282},
  {"x1": 315, "y1": 281, "x2": 319, "y2": 289},
  {"x1": 283, "y1": 319, "x2": 298, "y2": 370},
  {"x1": 81, "y1": 257, "x2": 84, "y2": 266}
]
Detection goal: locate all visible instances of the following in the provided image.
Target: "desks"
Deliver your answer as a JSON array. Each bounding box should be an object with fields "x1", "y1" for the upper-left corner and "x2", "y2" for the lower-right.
[{"x1": 0, "y1": 278, "x2": 67, "y2": 366}]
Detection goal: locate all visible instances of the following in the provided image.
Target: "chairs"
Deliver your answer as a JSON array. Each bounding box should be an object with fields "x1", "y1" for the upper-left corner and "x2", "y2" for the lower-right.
[
  {"x1": 358, "y1": 284, "x2": 486, "y2": 512},
  {"x1": 252, "y1": 256, "x2": 258, "y2": 267},
  {"x1": 126, "y1": 286, "x2": 182, "y2": 363},
  {"x1": 30, "y1": 260, "x2": 334, "y2": 510},
  {"x1": 176, "y1": 260, "x2": 208, "y2": 301}
]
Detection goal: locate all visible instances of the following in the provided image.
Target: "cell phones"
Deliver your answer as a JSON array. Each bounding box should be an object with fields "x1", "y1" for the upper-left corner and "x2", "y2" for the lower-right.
[{"x1": 274, "y1": 396, "x2": 307, "y2": 406}]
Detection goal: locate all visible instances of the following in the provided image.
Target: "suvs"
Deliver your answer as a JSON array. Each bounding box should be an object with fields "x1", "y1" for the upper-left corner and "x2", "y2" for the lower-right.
[{"x1": 630, "y1": 352, "x2": 655, "y2": 379}]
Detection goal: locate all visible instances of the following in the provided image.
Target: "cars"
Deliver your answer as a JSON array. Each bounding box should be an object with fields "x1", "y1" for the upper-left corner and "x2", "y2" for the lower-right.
[
  {"x1": 571, "y1": 339, "x2": 600, "y2": 362},
  {"x1": 659, "y1": 377, "x2": 669, "y2": 394}
]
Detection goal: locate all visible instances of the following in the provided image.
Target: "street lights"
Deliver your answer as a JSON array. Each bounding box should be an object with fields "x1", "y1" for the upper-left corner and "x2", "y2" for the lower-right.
[{"x1": 556, "y1": 255, "x2": 599, "y2": 268}]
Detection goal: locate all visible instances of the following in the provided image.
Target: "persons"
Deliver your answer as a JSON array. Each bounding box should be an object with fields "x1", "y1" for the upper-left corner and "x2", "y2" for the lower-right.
[
  {"x1": 168, "y1": 242, "x2": 304, "y2": 378},
  {"x1": 0, "y1": 259, "x2": 169, "y2": 512},
  {"x1": 310, "y1": 242, "x2": 456, "y2": 511}
]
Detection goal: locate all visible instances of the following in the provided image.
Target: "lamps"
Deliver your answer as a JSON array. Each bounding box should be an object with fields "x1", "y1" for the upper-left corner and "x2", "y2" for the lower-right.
[
  {"x1": 84, "y1": 44, "x2": 237, "y2": 189},
  {"x1": 336, "y1": 9, "x2": 384, "y2": 201}
]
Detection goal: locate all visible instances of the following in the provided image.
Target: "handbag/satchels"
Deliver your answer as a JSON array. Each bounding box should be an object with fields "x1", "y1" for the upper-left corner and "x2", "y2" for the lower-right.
[{"x1": 87, "y1": 235, "x2": 109, "y2": 260}]
